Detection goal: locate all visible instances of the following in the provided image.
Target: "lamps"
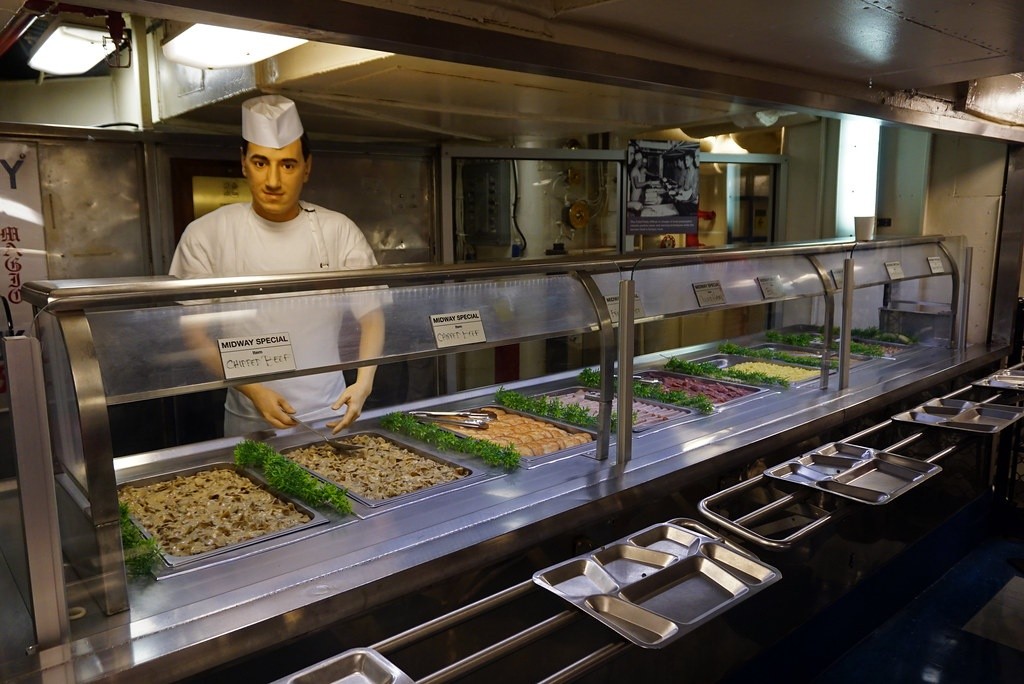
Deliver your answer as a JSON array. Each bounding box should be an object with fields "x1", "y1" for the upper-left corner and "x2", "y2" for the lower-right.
[
  {"x1": 160, "y1": 19, "x2": 310, "y2": 69},
  {"x1": 26, "y1": 9, "x2": 127, "y2": 76}
]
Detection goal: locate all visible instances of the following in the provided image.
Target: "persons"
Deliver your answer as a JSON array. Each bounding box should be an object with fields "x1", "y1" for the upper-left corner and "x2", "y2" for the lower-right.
[
  {"x1": 630, "y1": 148, "x2": 700, "y2": 215},
  {"x1": 168, "y1": 94, "x2": 393, "y2": 439}
]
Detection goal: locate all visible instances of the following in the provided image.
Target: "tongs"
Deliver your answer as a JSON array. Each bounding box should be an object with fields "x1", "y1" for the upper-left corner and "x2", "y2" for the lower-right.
[
  {"x1": 409, "y1": 410, "x2": 497, "y2": 429},
  {"x1": 639, "y1": 377, "x2": 665, "y2": 386}
]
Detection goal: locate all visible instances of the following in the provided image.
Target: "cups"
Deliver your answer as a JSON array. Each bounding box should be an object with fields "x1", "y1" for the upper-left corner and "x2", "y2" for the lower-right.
[{"x1": 854, "y1": 217, "x2": 874, "y2": 240}]
